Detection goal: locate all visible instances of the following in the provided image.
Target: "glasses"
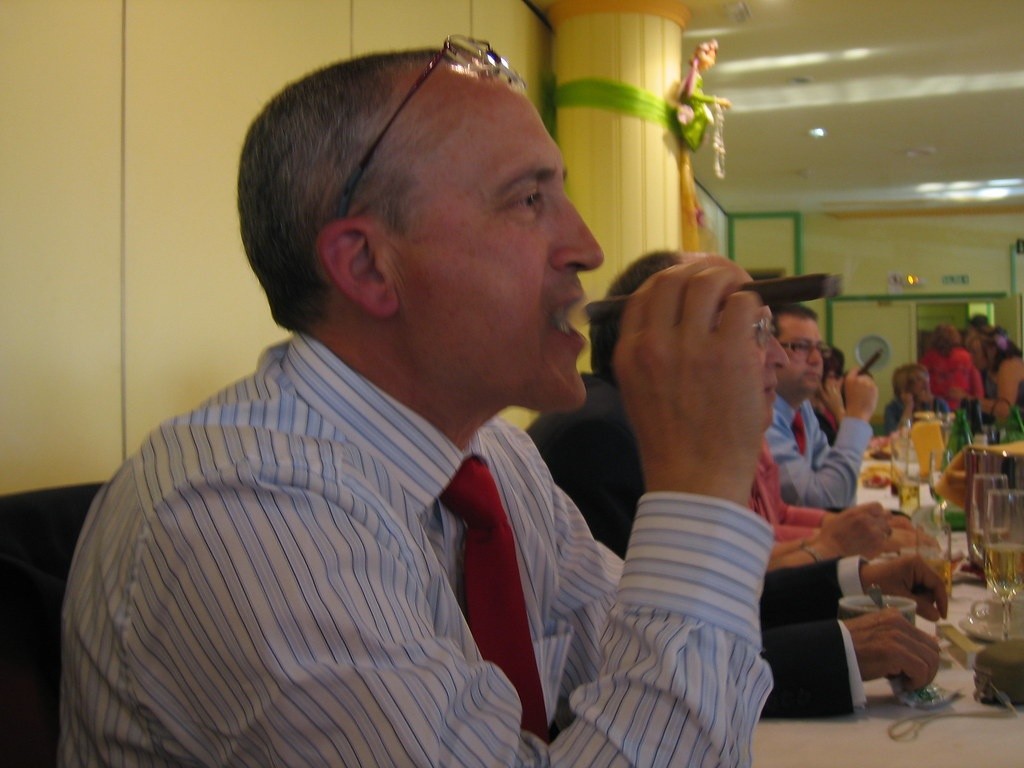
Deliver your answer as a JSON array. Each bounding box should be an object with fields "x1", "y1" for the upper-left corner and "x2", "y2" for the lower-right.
[
  {"x1": 752, "y1": 318, "x2": 769, "y2": 351},
  {"x1": 341, "y1": 33, "x2": 525, "y2": 220},
  {"x1": 783, "y1": 337, "x2": 832, "y2": 358}
]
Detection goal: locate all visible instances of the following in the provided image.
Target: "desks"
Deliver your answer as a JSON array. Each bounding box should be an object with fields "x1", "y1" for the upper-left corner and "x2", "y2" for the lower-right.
[{"x1": 750, "y1": 461, "x2": 1024, "y2": 768}]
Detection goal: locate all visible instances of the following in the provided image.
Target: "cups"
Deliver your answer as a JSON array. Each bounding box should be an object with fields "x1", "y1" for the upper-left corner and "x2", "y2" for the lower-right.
[
  {"x1": 890, "y1": 437, "x2": 920, "y2": 519},
  {"x1": 969, "y1": 473, "x2": 1009, "y2": 560},
  {"x1": 916, "y1": 521, "x2": 952, "y2": 597},
  {"x1": 836, "y1": 594, "x2": 916, "y2": 627}
]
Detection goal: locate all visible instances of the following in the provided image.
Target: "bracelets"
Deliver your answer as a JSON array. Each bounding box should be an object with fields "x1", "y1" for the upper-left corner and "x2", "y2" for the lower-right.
[{"x1": 802, "y1": 544, "x2": 823, "y2": 562}]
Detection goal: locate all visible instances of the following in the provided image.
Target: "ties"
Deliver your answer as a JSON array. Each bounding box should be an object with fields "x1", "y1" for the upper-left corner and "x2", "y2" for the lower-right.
[
  {"x1": 791, "y1": 410, "x2": 807, "y2": 455},
  {"x1": 437, "y1": 456, "x2": 549, "y2": 745}
]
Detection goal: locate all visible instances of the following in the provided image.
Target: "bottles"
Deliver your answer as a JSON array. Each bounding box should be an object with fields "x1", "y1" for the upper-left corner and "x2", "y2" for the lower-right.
[
  {"x1": 1001, "y1": 406, "x2": 1024, "y2": 489},
  {"x1": 943, "y1": 408, "x2": 974, "y2": 531}
]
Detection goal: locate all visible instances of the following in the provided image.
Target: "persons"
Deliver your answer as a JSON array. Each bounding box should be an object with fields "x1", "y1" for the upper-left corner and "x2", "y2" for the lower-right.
[
  {"x1": 527, "y1": 247, "x2": 1024, "y2": 719},
  {"x1": 60, "y1": 33, "x2": 772, "y2": 768},
  {"x1": 671, "y1": 39, "x2": 731, "y2": 227}
]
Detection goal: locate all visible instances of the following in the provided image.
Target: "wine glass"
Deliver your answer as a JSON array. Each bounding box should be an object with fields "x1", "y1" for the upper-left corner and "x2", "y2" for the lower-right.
[
  {"x1": 983, "y1": 490, "x2": 1024, "y2": 640},
  {"x1": 930, "y1": 451, "x2": 951, "y2": 530}
]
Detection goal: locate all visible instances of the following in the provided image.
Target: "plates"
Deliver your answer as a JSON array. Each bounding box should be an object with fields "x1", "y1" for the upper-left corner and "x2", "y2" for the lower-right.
[{"x1": 959, "y1": 615, "x2": 1024, "y2": 642}]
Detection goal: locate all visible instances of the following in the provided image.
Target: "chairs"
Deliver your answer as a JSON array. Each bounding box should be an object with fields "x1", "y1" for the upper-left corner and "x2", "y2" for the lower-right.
[{"x1": 0, "y1": 480, "x2": 105, "y2": 768}]
